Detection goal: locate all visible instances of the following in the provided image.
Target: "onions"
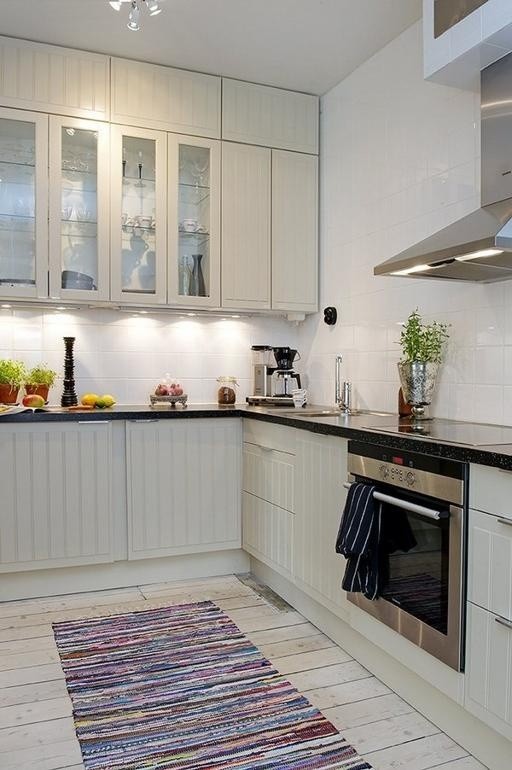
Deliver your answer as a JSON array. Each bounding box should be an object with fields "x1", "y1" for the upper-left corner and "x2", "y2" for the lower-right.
[{"x1": 155, "y1": 383, "x2": 184, "y2": 396}]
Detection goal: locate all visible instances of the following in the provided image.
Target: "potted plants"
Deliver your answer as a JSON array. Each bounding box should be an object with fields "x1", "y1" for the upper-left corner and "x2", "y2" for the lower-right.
[
  {"x1": 24, "y1": 367, "x2": 57, "y2": 402},
  {"x1": 392, "y1": 310, "x2": 452, "y2": 421},
  {"x1": 0, "y1": 359, "x2": 25, "y2": 403}
]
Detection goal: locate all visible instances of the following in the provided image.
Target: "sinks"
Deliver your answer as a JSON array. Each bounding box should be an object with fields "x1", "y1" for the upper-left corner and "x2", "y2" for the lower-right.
[{"x1": 295, "y1": 413, "x2": 358, "y2": 418}]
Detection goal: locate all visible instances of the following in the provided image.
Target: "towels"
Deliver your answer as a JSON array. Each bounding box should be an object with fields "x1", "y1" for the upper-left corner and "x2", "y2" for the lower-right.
[
  {"x1": 386, "y1": 500, "x2": 414, "y2": 561},
  {"x1": 331, "y1": 482, "x2": 378, "y2": 600}
]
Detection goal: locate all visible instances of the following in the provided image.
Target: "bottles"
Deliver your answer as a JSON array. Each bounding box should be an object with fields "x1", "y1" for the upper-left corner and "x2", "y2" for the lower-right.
[
  {"x1": 217, "y1": 376, "x2": 239, "y2": 406},
  {"x1": 61, "y1": 337, "x2": 80, "y2": 407},
  {"x1": 179, "y1": 254, "x2": 195, "y2": 296},
  {"x1": 191, "y1": 253, "x2": 206, "y2": 296}
]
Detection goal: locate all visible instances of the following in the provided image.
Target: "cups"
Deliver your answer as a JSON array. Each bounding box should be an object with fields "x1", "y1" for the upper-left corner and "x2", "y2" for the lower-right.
[
  {"x1": 135, "y1": 215, "x2": 156, "y2": 227},
  {"x1": 292, "y1": 397, "x2": 308, "y2": 408},
  {"x1": 61, "y1": 202, "x2": 73, "y2": 219},
  {"x1": 121, "y1": 213, "x2": 133, "y2": 225},
  {"x1": 182, "y1": 219, "x2": 210, "y2": 233},
  {"x1": 61, "y1": 149, "x2": 95, "y2": 173},
  {"x1": 73, "y1": 206, "x2": 93, "y2": 221},
  {"x1": 292, "y1": 389, "x2": 307, "y2": 399}
]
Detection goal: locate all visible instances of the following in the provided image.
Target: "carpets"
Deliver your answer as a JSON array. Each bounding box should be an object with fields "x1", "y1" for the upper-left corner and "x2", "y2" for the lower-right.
[
  {"x1": 49, "y1": 598, "x2": 373, "y2": 769},
  {"x1": 386, "y1": 570, "x2": 446, "y2": 625}
]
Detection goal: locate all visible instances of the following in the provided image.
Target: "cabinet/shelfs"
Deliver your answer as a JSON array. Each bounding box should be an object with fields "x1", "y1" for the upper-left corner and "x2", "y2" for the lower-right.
[
  {"x1": 467, "y1": 462, "x2": 512, "y2": 729},
  {"x1": 2, "y1": 32, "x2": 108, "y2": 309},
  {"x1": 296, "y1": 428, "x2": 350, "y2": 618},
  {"x1": 1, "y1": 418, "x2": 243, "y2": 578},
  {"x1": 243, "y1": 416, "x2": 297, "y2": 592},
  {"x1": 223, "y1": 72, "x2": 320, "y2": 321},
  {"x1": 109, "y1": 53, "x2": 221, "y2": 316}
]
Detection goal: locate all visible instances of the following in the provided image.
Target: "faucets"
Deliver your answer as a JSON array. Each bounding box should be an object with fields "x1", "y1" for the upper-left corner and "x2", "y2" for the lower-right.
[{"x1": 335, "y1": 356, "x2": 349, "y2": 412}]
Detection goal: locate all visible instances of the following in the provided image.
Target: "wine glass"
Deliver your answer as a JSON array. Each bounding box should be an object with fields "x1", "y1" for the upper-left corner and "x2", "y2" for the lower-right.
[{"x1": 178, "y1": 155, "x2": 207, "y2": 192}]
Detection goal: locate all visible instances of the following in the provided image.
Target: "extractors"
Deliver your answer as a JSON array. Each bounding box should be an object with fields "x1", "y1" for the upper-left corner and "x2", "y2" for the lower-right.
[{"x1": 370, "y1": 51, "x2": 511, "y2": 285}]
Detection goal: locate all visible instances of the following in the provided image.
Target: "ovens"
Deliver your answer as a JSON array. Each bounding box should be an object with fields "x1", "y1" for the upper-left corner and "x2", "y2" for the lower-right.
[{"x1": 337, "y1": 438, "x2": 470, "y2": 676}]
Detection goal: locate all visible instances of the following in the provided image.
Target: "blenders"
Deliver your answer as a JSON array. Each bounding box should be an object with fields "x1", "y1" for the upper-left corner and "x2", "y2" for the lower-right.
[{"x1": 250, "y1": 345, "x2": 273, "y2": 396}]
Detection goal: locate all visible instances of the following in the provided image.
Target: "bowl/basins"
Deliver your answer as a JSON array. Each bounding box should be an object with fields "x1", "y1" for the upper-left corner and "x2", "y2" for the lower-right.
[{"x1": 62, "y1": 269, "x2": 94, "y2": 289}]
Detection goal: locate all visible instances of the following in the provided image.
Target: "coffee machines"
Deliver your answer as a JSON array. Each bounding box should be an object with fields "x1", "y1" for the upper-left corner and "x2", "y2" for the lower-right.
[{"x1": 246, "y1": 343, "x2": 303, "y2": 405}]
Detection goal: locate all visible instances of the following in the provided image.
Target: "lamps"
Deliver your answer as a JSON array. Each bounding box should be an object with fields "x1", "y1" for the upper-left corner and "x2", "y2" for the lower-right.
[{"x1": 108, "y1": 0, "x2": 165, "y2": 30}]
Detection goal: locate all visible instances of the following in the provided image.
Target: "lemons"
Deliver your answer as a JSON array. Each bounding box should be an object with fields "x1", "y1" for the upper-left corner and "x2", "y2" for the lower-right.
[{"x1": 81, "y1": 393, "x2": 116, "y2": 408}]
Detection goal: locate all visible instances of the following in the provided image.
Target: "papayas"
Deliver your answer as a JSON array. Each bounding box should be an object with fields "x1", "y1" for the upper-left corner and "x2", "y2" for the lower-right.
[{"x1": 23, "y1": 394, "x2": 45, "y2": 408}]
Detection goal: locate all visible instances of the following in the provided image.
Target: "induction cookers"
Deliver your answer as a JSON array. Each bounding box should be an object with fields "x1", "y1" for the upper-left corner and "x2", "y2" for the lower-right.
[{"x1": 365, "y1": 421, "x2": 511, "y2": 449}]
